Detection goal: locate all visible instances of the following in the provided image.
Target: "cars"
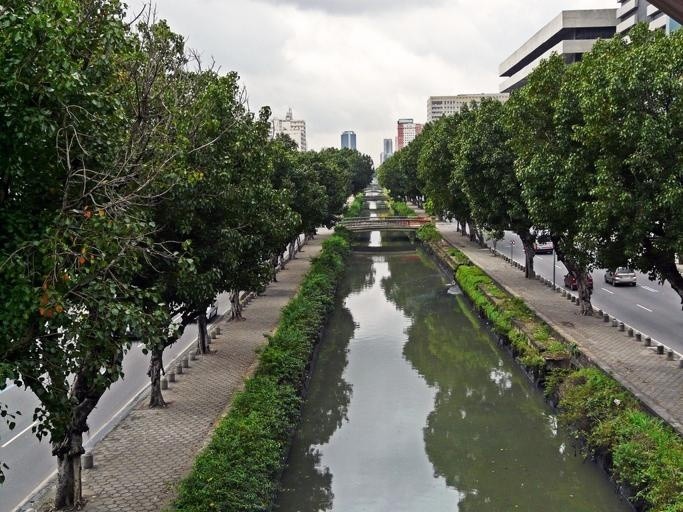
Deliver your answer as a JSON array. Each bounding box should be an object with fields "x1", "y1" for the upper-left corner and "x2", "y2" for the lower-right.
[
  {"x1": 604, "y1": 265, "x2": 637, "y2": 287},
  {"x1": 189, "y1": 303, "x2": 218, "y2": 321},
  {"x1": 562, "y1": 271, "x2": 593, "y2": 293},
  {"x1": 534, "y1": 234, "x2": 555, "y2": 253}
]
